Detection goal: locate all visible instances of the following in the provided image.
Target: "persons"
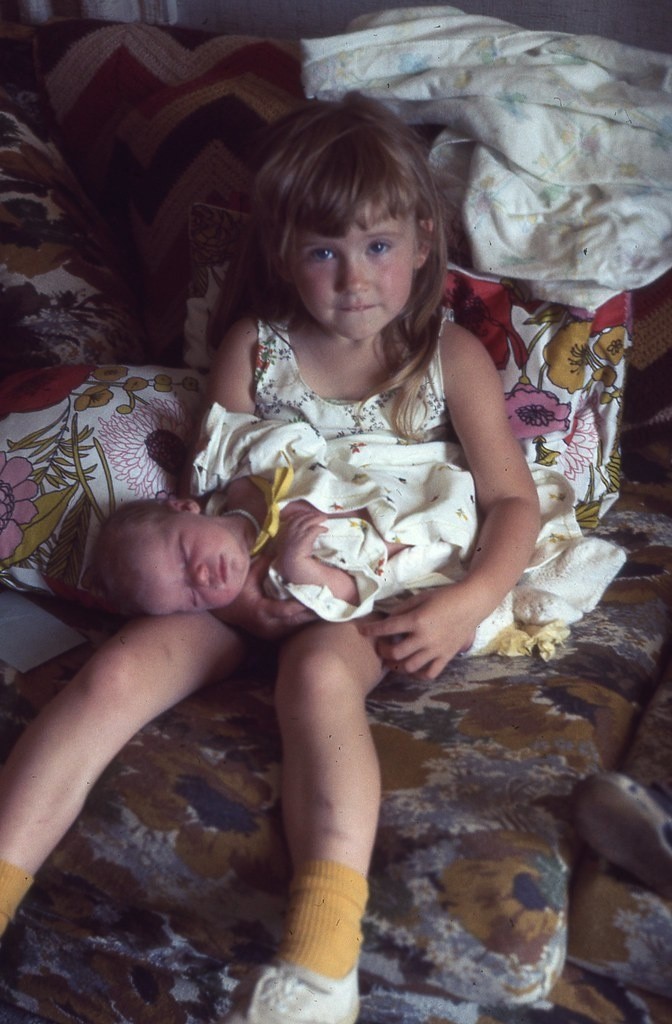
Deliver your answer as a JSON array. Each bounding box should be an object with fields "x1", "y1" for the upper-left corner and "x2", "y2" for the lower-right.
[
  {"x1": 0, "y1": 103, "x2": 539, "y2": 1024},
  {"x1": 87, "y1": 399, "x2": 586, "y2": 619}
]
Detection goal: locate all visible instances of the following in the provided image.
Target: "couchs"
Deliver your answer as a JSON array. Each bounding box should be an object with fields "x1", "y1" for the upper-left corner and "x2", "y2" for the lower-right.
[{"x1": 0, "y1": 0, "x2": 672, "y2": 1024}]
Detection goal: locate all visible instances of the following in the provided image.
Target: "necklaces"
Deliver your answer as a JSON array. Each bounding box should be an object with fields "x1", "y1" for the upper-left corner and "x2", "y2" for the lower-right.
[{"x1": 220, "y1": 509, "x2": 261, "y2": 538}]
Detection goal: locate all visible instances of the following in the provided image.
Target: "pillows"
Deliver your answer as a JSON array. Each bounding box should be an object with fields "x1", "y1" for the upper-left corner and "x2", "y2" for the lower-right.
[
  {"x1": 0, "y1": 361, "x2": 210, "y2": 615},
  {"x1": 441, "y1": 260, "x2": 634, "y2": 531},
  {"x1": 0, "y1": 81, "x2": 148, "y2": 374}
]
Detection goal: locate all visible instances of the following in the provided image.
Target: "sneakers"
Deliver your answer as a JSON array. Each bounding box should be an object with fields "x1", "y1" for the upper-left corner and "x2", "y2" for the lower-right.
[{"x1": 225, "y1": 950, "x2": 362, "y2": 1024}]
